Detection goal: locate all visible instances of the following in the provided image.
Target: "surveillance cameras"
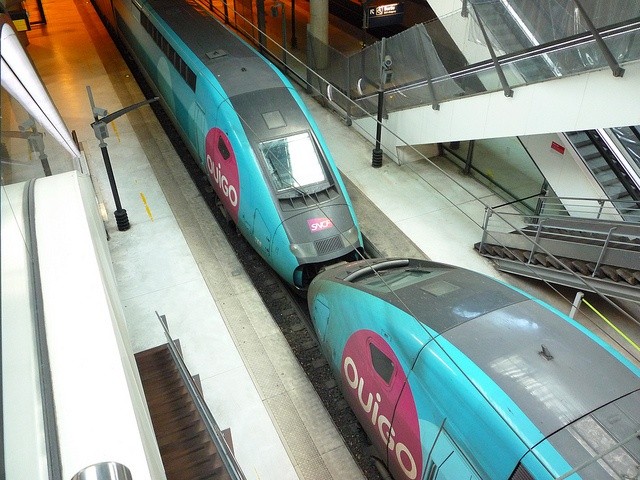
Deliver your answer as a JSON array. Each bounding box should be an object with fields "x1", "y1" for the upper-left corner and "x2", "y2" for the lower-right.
[
  {"x1": 93, "y1": 107, "x2": 109, "y2": 119},
  {"x1": 383, "y1": 55, "x2": 393, "y2": 69}
]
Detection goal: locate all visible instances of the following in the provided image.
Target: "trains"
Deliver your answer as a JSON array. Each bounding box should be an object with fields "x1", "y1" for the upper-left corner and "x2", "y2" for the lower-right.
[
  {"x1": 89, "y1": 1, "x2": 365, "y2": 291},
  {"x1": 307, "y1": 257, "x2": 640, "y2": 476}
]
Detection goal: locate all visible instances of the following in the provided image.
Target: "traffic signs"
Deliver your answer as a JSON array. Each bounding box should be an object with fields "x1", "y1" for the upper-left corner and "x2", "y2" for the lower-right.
[{"x1": 366, "y1": 2, "x2": 402, "y2": 18}]
